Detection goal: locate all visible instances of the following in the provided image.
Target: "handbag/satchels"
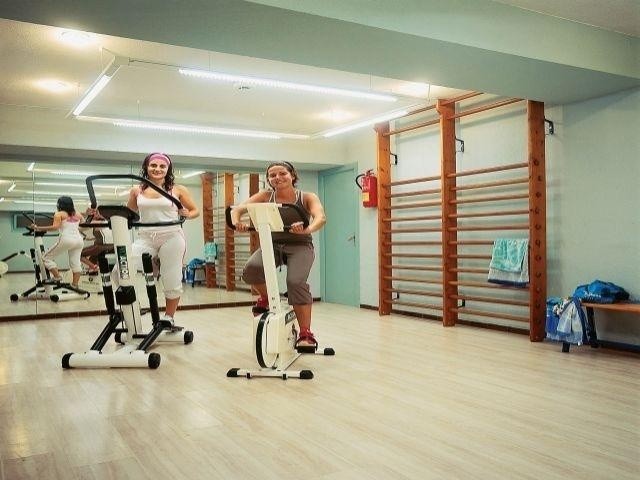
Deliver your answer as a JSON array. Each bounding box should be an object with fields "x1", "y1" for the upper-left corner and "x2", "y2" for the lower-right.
[{"x1": 572, "y1": 280, "x2": 630, "y2": 305}]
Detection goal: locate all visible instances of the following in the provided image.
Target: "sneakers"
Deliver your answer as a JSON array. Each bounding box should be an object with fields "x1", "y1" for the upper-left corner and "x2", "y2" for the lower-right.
[
  {"x1": 45, "y1": 276, "x2": 61, "y2": 283},
  {"x1": 296, "y1": 327, "x2": 315, "y2": 347},
  {"x1": 64, "y1": 282, "x2": 78, "y2": 291},
  {"x1": 160, "y1": 314, "x2": 175, "y2": 327},
  {"x1": 253, "y1": 297, "x2": 269, "y2": 317}
]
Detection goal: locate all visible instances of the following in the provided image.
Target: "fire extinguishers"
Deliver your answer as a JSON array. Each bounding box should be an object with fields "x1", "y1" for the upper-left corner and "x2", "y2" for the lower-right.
[{"x1": 355, "y1": 168, "x2": 377, "y2": 207}]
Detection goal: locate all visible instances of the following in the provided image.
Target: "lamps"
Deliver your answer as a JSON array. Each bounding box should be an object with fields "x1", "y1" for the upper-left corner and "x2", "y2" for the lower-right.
[
  {"x1": 177, "y1": 67, "x2": 399, "y2": 106},
  {"x1": 323, "y1": 109, "x2": 409, "y2": 139},
  {"x1": 73, "y1": 71, "x2": 113, "y2": 118},
  {"x1": 0, "y1": 161, "x2": 207, "y2": 206},
  {"x1": 108, "y1": 118, "x2": 283, "y2": 141}
]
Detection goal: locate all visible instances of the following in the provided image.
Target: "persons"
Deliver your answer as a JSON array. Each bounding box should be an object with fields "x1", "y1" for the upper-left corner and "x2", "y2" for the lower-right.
[
  {"x1": 230, "y1": 160, "x2": 326, "y2": 347},
  {"x1": 86, "y1": 152, "x2": 200, "y2": 328},
  {"x1": 80, "y1": 207, "x2": 114, "y2": 275},
  {"x1": 30, "y1": 196, "x2": 86, "y2": 289}
]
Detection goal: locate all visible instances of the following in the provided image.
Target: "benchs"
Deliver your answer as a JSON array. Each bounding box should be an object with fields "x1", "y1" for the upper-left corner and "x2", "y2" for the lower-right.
[{"x1": 560, "y1": 300, "x2": 640, "y2": 353}]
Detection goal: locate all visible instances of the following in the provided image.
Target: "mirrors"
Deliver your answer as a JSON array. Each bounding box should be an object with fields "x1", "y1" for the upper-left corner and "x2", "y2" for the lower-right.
[{"x1": 0, "y1": 158, "x2": 280, "y2": 317}]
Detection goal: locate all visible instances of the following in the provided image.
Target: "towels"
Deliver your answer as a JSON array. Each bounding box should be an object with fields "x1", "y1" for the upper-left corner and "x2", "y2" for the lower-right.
[
  {"x1": 202, "y1": 242, "x2": 218, "y2": 263},
  {"x1": 486, "y1": 238, "x2": 530, "y2": 289}
]
